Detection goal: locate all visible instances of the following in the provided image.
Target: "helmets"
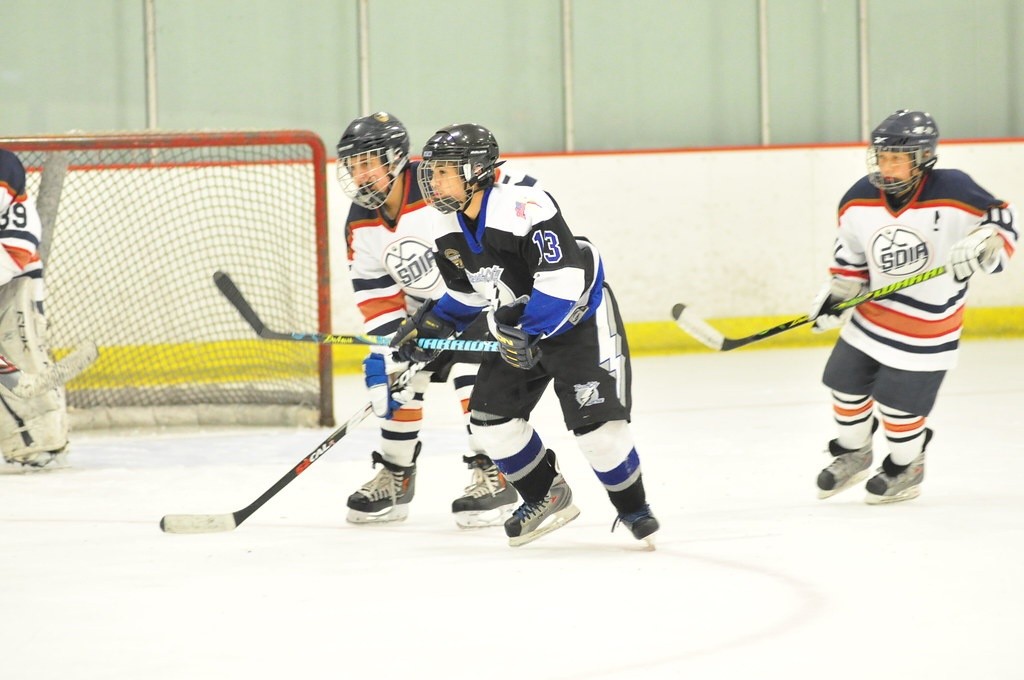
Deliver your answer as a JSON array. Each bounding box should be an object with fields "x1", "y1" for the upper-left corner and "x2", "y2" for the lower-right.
[
  {"x1": 336, "y1": 112, "x2": 410, "y2": 211},
  {"x1": 866, "y1": 108, "x2": 938, "y2": 196},
  {"x1": 417, "y1": 124, "x2": 499, "y2": 215}
]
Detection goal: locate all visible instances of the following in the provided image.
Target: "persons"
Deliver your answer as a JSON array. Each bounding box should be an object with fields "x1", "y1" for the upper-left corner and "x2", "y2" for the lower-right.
[
  {"x1": 0, "y1": 147, "x2": 72, "y2": 470},
  {"x1": 389, "y1": 124, "x2": 661, "y2": 555},
  {"x1": 337, "y1": 113, "x2": 544, "y2": 531},
  {"x1": 815, "y1": 111, "x2": 1019, "y2": 505}
]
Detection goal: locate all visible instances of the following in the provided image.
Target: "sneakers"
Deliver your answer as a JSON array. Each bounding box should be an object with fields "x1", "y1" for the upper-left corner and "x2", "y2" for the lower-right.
[
  {"x1": 344, "y1": 439, "x2": 423, "y2": 523},
  {"x1": 611, "y1": 506, "x2": 661, "y2": 551},
  {"x1": 452, "y1": 454, "x2": 520, "y2": 526},
  {"x1": 504, "y1": 450, "x2": 581, "y2": 546},
  {"x1": 816, "y1": 416, "x2": 881, "y2": 501},
  {"x1": 864, "y1": 426, "x2": 933, "y2": 504}
]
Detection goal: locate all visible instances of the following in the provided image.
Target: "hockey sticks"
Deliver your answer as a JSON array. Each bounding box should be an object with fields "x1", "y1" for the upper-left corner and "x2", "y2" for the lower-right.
[
  {"x1": 158, "y1": 362, "x2": 424, "y2": 535},
  {"x1": 0, "y1": 338, "x2": 100, "y2": 399},
  {"x1": 213, "y1": 270, "x2": 538, "y2": 359},
  {"x1": 670, "y1": 260, "x2": 953, "y2": 352}
]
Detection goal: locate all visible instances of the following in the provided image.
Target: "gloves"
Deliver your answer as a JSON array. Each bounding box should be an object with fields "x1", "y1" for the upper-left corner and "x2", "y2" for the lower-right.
[
  {"x1": 389, "y1": 298, "x2": 457, "y2": 362},
  {"x1": 812, "y1": 275, "x2": 867, "y2": 334},
  {"x1": 364, "y1": 353, "x2": 416, "y2": 421},
  {"x1": 951, "y1": 224, "x2": 1015, "y2": 284},
  {"x1": 495, "y1": 325, "x2": 543, "y2": 370}
]
